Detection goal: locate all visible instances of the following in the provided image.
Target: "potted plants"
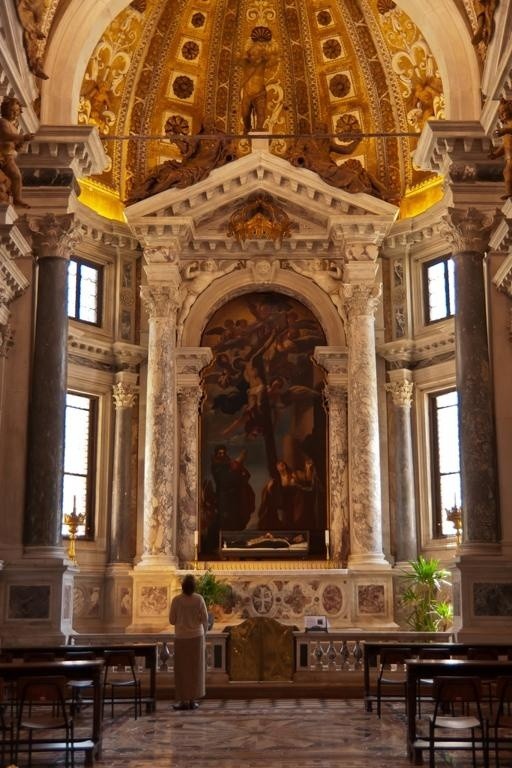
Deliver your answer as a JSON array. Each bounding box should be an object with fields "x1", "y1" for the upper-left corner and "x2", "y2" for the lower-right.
[{"x1": 193, "y1": 567, "x2": 230, "y2": 631}]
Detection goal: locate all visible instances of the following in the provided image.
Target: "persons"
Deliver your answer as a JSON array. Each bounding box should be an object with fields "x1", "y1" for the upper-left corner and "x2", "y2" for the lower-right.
[
  {"x1": 86, "y1": 81, "x2": 115, "y2": 123},
  {"x1": 232, "y1": 42, "x2": 281, "y2": 136},
  {"x1": 484, "y1": 103, "x2": 512, "y2": 200},
  {"x1": 128, "y1": 116, "x2": 240, "y2": 200},
  {"x1": 197, "y1": 300, "x2": 327, "y2": 559},
  {"x1": 0, "y1": 96, "x2": 38, "y2": 210},
  {"x1": 292, "y1": 118, "x2": 388, "y2": 198},
  {"x1": 414, "y1": 83, "x2": 441, "y2": 125},
  {"x1": 167, "y1": 574, "x2": 211, "y2": 711}
]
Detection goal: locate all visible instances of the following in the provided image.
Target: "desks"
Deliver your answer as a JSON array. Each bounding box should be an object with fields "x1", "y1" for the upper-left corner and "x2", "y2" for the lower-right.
[
  {"x1": 2, "y1": 643, "x2": 160, "y2": 715},
  {"x1": 362, "y1": 641, "x2": 511, "y2": 712}
]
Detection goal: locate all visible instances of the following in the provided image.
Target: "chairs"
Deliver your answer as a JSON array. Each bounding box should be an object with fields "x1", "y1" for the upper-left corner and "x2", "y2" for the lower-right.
[
  {"x1": 0, "y1": 650, "x2": 142, "y2": 768},
  {"x1": 375, "y1": 646, "x2": 511, "y2": 768}
]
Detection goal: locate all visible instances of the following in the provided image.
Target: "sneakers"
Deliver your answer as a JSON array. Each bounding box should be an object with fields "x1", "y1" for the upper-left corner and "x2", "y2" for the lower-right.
[
  {"x1": 191, "y1": 702, "x2": 199, "y2": 709},
  {"x1": 172, "y1": 703, "x2": 189, "y2": 710}
]
